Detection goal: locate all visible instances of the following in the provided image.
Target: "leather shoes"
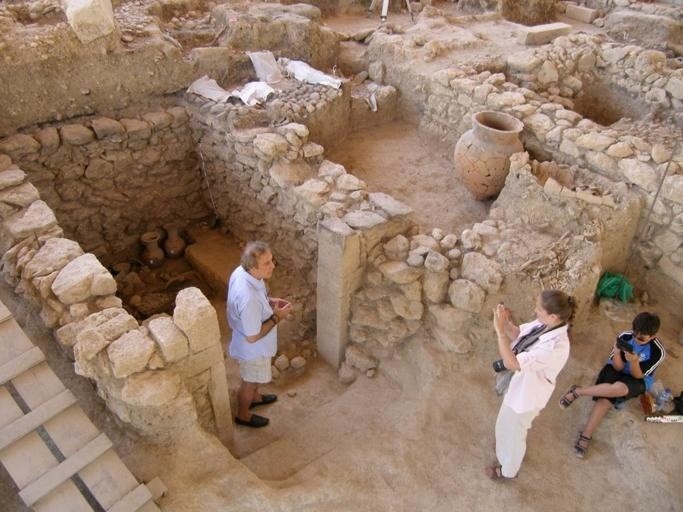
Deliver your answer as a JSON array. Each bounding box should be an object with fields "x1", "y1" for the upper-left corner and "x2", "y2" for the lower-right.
[
  {"x1": 250, "y1": 393, "x2": 276, "y2": 410},
  {"x1": 235, "y1": 412, "x2": 269, "y2": 428}
]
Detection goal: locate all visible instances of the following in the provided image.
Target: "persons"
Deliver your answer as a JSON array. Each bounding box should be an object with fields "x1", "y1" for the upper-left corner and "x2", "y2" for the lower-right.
[
  {"x1": 225, "y1": 240, "x2": 293, "y2": 428},
  {"x1": 485, "y1": 289, "x2": 578, "y2": 482},
  {"x1": 558, "y1": 312, "x2": 666, "y2": 460}
]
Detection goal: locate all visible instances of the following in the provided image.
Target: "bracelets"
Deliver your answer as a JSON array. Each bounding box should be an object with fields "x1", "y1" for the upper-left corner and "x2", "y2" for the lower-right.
[{"x1": 271, "y1": 314, "x2": 279, "y2": 324}]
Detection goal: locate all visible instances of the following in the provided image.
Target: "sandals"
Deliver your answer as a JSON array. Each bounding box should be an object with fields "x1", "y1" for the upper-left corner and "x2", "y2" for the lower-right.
[
  {"x1": 559, "y1": 383, "x2": 582, "y2": 412},
  {"x1": 573, "y1": 430, "x2": 592, "y2": 458},
  {"x1": 485, "y1": 466, "x2": 518, "y2": 481}
]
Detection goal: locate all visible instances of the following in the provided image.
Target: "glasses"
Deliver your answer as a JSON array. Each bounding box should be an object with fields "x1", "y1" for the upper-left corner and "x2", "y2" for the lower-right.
[{"x1": 631, "y1": 332, "x2": 651, "y2": 342}]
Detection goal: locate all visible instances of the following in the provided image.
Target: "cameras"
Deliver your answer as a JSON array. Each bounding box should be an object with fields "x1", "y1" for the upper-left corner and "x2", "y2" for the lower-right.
[{"x1": 493, "y1": 360, "x2": 506, "y2": 373}]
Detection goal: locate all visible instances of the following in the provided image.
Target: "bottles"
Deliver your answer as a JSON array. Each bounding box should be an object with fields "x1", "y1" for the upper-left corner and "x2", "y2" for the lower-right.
[
  {"x1": 140, "y1": 222, "x2": 185, "y2": 268},
  {"x1": 657, "y1": 387, "x2": 671, "y2": 412}
]
